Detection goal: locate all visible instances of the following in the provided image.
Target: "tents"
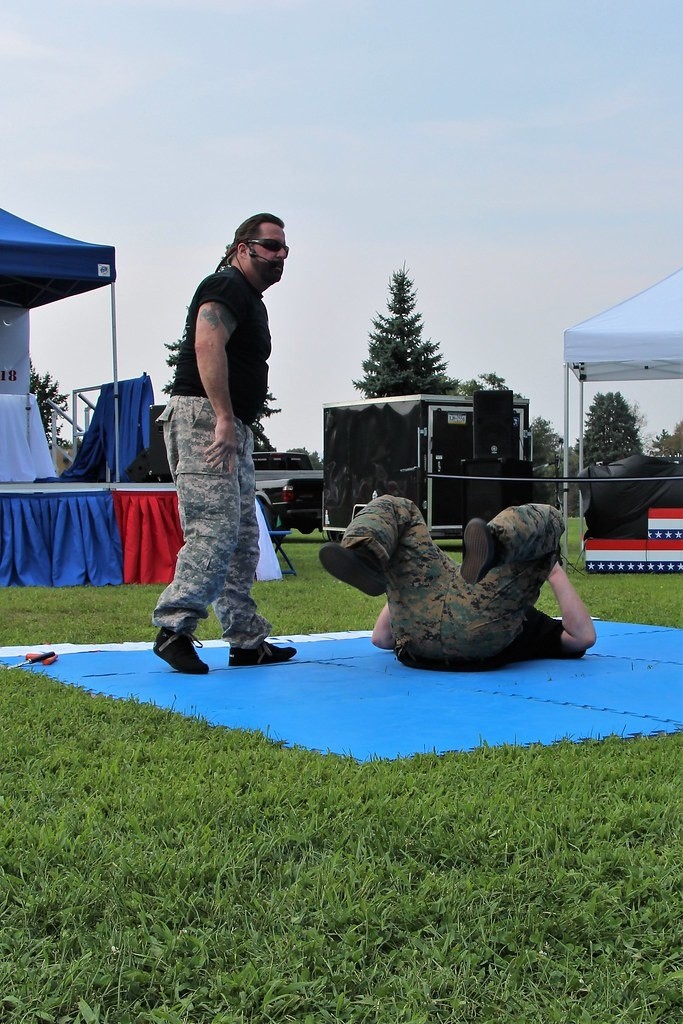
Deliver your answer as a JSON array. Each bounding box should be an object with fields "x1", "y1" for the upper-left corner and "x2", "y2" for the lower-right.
[
  {"x1": 563, "y1": 266, "x2": 683, "y2": 575},
  {"x1": 1, "y1": 209, "x2": 120, "y2": 484}
]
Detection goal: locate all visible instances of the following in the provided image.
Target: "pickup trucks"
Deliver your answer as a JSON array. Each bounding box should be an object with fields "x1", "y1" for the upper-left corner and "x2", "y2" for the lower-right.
[{"x1": 252, "y1": 451, "x2": 326, "y2": 539}]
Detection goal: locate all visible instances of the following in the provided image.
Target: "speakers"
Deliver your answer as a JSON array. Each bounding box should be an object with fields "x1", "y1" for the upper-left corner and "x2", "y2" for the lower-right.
[
  {"x1": 473, "y1": 390, "x2": 515, "y2": 459},
  {"x1": 124, "y1": 405, "x2": 174, "y2": 483}
]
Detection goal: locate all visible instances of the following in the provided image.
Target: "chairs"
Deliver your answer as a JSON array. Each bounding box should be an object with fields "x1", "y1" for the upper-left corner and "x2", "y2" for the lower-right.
[{"x1": 256, "y1": 496, "x2": 297, "y2": 577}]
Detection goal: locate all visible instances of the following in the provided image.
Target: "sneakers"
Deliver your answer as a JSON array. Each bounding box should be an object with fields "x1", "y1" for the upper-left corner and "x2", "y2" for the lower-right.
[
  {"x1": 228, "y1": 640, "x2": 297, "y2": 666},
  {"x1": 154, "y1": 625, "x2": 209, "y2": 674}
]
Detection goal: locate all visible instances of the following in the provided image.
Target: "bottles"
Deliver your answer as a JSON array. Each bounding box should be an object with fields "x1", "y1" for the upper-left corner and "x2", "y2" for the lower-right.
[
  {"x1": 372, "y1": 490, "x2": 377, "y2": 500},
  {"x1": 325, "y1": 509, "x2": 329, "y2": 527}
]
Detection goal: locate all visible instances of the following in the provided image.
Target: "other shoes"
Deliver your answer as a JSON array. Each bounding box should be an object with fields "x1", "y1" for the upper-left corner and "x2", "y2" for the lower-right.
[
  {"x1": 319, "y1": 544, "x2": 386, "y2": 597},
  {"x1": 461, "y1": 518, "x2": 495, "y2": 585}
]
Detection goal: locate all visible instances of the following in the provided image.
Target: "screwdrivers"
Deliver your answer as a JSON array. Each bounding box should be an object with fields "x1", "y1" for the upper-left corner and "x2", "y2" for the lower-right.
[{"x1": 7, "y1": 651, "x2": 55, "y2": 670}]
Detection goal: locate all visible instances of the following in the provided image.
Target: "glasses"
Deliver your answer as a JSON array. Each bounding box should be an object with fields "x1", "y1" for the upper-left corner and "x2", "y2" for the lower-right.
[{"x1": 247, "y1": 239, "x2": 289, "y2": 259}]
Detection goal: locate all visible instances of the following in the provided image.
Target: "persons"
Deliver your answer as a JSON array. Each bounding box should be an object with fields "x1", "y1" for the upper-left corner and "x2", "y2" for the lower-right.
[
  {"x1": 320, "y1": 494, "x2": 597, "y2": 675},
  {"x1": 150, "y1": 215, "x2": 297, "y2": 673}
]
